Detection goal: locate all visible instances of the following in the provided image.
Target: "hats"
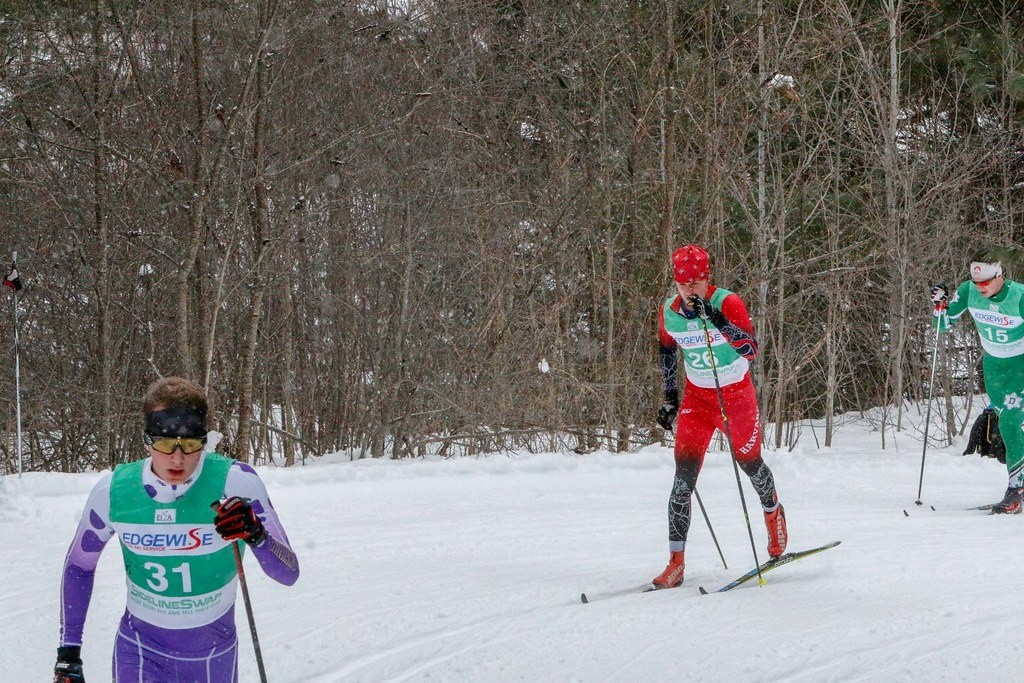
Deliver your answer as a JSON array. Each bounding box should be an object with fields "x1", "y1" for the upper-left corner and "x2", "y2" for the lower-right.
[{"x1": 673, "y1": 244, "x2": 710, "y2": 283}]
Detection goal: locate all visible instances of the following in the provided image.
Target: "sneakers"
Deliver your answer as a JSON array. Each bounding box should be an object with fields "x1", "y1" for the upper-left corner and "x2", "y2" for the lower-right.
[
  {"x1": 991, "y1": 487, "x2": 1024, "y2": 516},
  {"x1": 652, "y1": 551, "x2": 685, "y2": 588},
  {"x1": 764, "y1": 502, "x2": 788, "y2": 557}
]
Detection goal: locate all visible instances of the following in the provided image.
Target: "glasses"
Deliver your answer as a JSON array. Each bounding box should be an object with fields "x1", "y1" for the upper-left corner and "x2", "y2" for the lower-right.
[
  {"x1": 972, "y1": 278, "x2": 994, "y2": 287},
  {"x1": 147, "y1": 435, "x2": 207, "y2": 455}
]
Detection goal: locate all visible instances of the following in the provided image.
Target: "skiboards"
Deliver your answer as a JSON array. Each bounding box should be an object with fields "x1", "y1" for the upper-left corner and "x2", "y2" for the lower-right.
[
  {"x1": 579, "y1": 540, "x2": 844, "y2": 605},
  {"x1": 902, "y1": 504, "x2": 1016, "y2": 519}
]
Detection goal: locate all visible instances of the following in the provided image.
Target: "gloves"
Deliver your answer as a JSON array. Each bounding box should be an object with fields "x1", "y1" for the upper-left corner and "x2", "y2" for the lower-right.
[
  {"x1": 930, "y1": 278, "x2": 950, "y2": 311},
  {"x1": 687, "y1": 294, "x2": 717, "y2": 323},
  {"x1": 52, "y1": 646, "x2": 86, "y2": 683},
  {"x1": 656, "y1": 399, "x2": 678, "y2": 430},
  {"x1": 210, "y1": 496, "x2": 269, "y2": 547}
]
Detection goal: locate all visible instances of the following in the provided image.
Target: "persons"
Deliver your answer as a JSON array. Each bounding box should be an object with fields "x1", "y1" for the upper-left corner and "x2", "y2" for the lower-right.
[
  {"x1": 930, "y1": 250, "x2": 1024, "y2": 515},
  {"x1": 52, "y1": 378, "x2": 300, "y2": 683},
  {"x1": 652, "y1": 246, "x2": 788, "y2": 589}
]
